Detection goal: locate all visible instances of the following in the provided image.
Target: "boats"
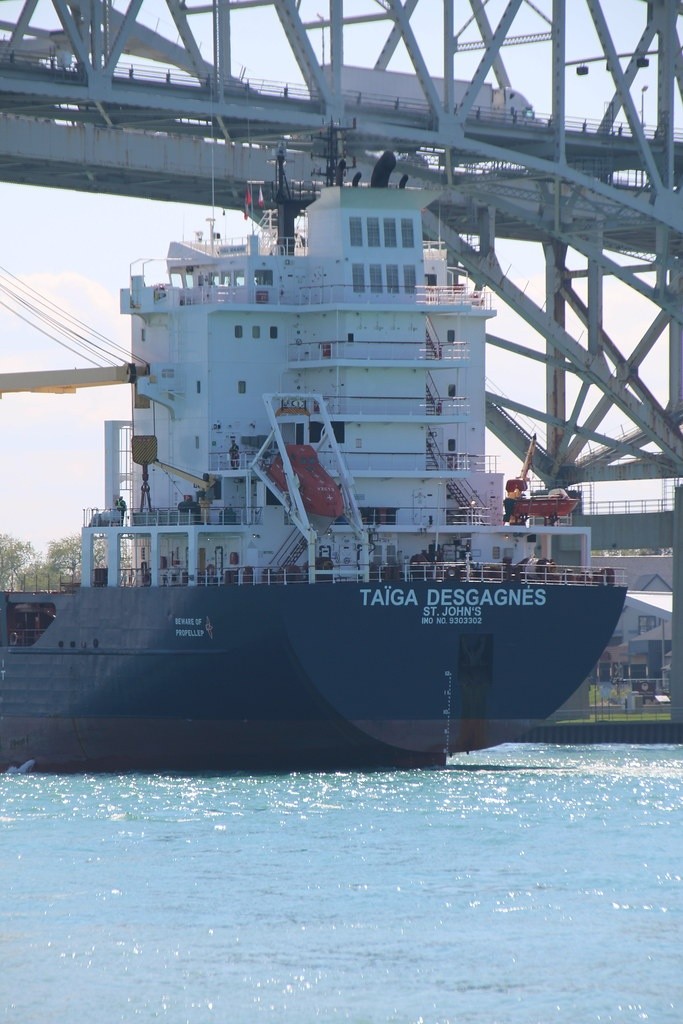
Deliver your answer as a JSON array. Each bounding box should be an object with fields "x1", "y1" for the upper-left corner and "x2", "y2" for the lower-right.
[
  {"x1": 271, "y1": 441, "x2": 347, "y2": 519},
  {"x1": 515, "y1": 496, "x2": 580, "y2": 519},
  {"x1": 0, "y1": 0, "x2": 633, "y2": 772}
]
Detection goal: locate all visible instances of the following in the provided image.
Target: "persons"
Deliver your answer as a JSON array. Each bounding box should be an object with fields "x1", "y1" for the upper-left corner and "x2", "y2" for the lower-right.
[
  {"x1": 229, "y1": 440, "x2": 240, "y2": 469},
  {"x1": 116, "y1": 497, "x2": 126, "y2": 526}
]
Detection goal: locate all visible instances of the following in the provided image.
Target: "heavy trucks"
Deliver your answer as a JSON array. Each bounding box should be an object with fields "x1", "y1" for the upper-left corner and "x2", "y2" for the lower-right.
[{"x1": 313, "y1": 63, "x2": 535, "y2": 123}]
